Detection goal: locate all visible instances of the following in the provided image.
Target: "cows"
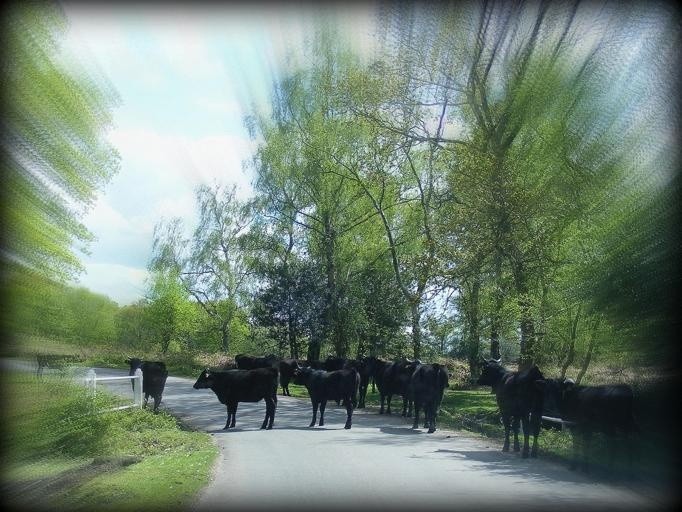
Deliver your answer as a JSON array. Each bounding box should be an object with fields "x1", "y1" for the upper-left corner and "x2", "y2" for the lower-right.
[
  {"x1": 124, "y1": 356, "x2": 168, "y2": 411},
  {"x1": 534, "y1": 378, "x2": 642, "y2": 478},
  {"x1": 192, "y1": 367, "x2": 278, "y2": 430},
  {"x1": 234, "y1": 353, "x2": 450, "y2": 434},
  {"x1": 37, "y1": 352, "x2": 87, "y2": 380},
  {"x1": 477, "y1": 354, "x2": 545, "y2": 459}
]
